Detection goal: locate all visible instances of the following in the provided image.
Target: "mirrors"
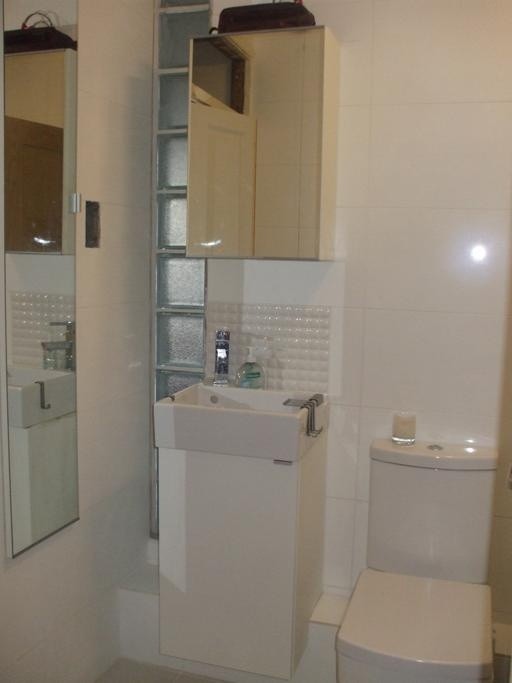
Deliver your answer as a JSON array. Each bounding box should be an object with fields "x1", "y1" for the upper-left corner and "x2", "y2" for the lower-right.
[{"x1": 0, "y1": 0, "x2": 77, "y2": 561}]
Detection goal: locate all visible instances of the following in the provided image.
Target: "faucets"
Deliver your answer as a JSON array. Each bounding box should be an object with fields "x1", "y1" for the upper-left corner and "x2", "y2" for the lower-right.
[
  {"x1": 211, "y1": 328, "x2": 229, "y2": 385},
  {"x1": 42, "y1": 318, "x2": 77, "y2": 370}
]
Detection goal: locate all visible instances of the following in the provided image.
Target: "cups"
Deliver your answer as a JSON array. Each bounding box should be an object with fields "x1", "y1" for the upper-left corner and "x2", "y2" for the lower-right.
[{"x1": 392, "y1": 411, "x2": 417, "y2": 446}]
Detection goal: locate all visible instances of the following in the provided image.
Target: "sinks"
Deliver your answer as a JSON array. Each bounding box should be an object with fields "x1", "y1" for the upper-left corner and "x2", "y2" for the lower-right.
[
  {"x1": 152, "y1": 382, "x2": 330, "y2": 463},
  {"x1": 7, "y1": 360, "x2": 76, "y2": 428}
]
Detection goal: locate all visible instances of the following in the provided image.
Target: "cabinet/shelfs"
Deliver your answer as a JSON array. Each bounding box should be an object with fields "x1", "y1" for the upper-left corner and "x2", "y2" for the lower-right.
[
  {"x1": 3, "y1": 46, "x2": 77, "y2": 256},
  {"x1": 184, "y1": 23, "x2": 342, "y2": 263}
]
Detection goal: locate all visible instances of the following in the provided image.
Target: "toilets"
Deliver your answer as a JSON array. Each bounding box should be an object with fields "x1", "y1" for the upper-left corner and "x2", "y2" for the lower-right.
[{"x1": 333, "y1": 437, "x2": 501, "y2": 683}]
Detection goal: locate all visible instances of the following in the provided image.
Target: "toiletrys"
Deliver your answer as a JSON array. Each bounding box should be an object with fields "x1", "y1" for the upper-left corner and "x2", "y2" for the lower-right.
[
  {"x1": 43, "y1": 347, "x2": 57, "y2": 371},
  {"x1": 234, "y1": 345, "x2": 265, "y2": 389}
]
